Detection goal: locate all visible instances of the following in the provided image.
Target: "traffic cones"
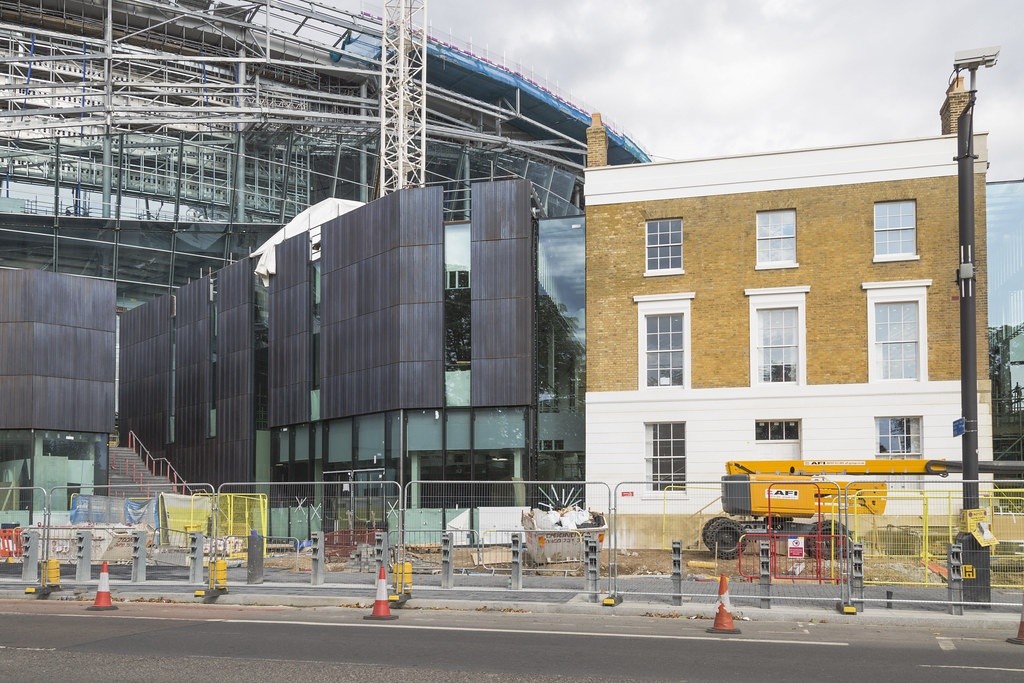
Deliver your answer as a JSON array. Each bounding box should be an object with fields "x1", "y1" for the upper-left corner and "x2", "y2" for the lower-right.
[
  {"x1": 364, "y1": 566, "x2": 400, "y2": 620},
  {"x1": 86, "y1": 560, "x2": 118, "y2": 611},
  {"x1": 1006, "y1": 594, "x2": 1024, "y2": 644},
  {"x1": 706, "y1": 573, "x2": 743, "y2": 634}
]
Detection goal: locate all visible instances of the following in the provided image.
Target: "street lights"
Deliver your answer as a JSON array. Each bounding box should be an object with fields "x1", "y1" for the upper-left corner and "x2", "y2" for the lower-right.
[{"x1": 943, "y1": 48, "x2": 1001, "y2": 608}]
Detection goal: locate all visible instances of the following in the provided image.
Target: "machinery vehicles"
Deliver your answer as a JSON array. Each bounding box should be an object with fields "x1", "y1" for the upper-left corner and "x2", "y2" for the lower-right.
[{"x1": 702, "y1": 459, "x2": 1024, "y2": 560}]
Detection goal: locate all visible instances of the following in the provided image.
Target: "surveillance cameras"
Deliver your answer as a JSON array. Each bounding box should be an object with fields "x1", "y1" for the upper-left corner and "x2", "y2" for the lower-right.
[{"x1": 953, "y1": 45, "x2": 1001, "y2": 70}]
